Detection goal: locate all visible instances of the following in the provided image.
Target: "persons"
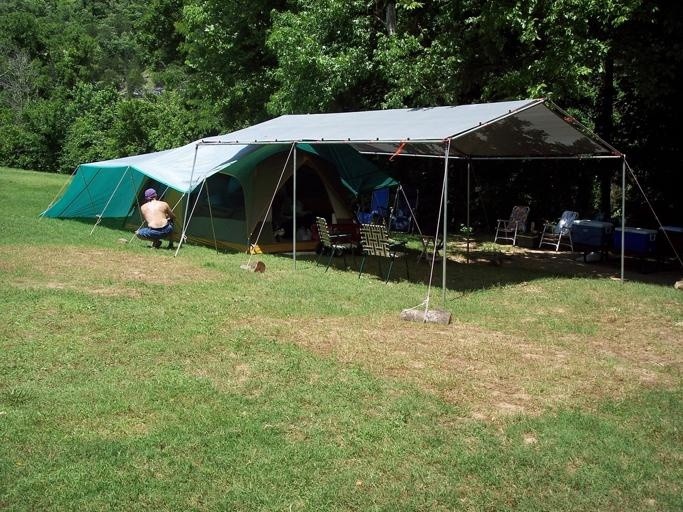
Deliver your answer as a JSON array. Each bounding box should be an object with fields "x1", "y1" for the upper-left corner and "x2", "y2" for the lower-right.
[{"x1": 137, "y1": 188, "x2": 177, "y2": 250}]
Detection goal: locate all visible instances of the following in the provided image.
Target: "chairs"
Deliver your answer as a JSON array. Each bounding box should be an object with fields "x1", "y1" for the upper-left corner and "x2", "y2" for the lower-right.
[
  {"x1": 494, "y1": 205, "x2": 530, "y2": 246},
  {"x1": 315, "y1": 217, "x2": 410, "y2": 287},
  {"x1": 539, "y1": 210, "x2": 579, "y2": 254}
]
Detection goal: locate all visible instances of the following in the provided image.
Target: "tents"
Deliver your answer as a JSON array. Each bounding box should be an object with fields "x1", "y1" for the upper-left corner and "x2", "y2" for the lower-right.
[{"x1": 128, "y1": 143, "x2": 400, "y2": 254}]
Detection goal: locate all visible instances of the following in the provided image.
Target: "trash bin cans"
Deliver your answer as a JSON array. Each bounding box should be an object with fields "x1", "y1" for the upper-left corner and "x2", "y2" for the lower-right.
[{"x1": 572, "y1": 220, "x2": 683, "y2": 254}]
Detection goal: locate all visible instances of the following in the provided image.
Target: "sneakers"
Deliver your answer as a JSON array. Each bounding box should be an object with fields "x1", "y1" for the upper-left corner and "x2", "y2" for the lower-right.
[{"x1": 152, "y1": 240, "x2": 174, "y2": 249}]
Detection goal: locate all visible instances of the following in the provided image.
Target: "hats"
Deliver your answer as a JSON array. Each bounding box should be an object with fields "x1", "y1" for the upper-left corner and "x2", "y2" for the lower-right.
[{"x1": 145, "y1": 188, "x2": 156, "y2": 200}]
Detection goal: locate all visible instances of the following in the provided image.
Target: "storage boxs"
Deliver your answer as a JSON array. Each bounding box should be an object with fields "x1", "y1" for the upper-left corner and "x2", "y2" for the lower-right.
[
  {"x1": 516, "y1": 234, "x2": 539, "y2": 248},
  {"x1": 573, "y1": 220, "x2": 683, "y2": 254}
]
